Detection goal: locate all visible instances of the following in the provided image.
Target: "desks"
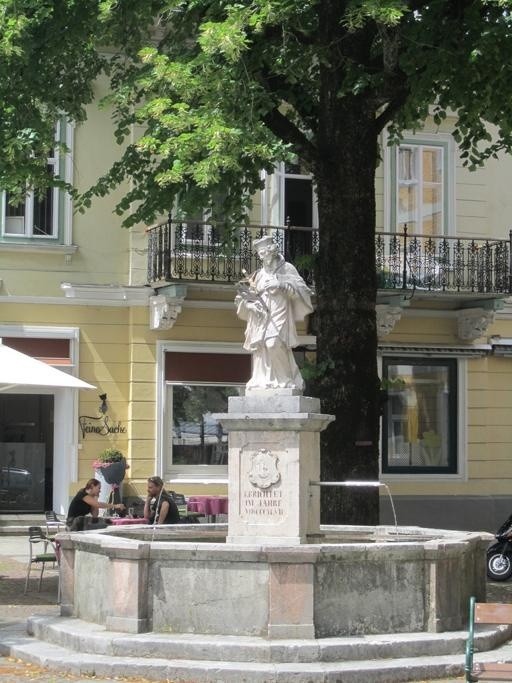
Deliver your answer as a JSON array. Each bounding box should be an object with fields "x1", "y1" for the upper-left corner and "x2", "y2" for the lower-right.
[
  {"x1": 188, "y1": 495, "x2": 228, "y2": 524},
  {"x1": 100, "y1": 516, "x2": 150, "y2": 526}
]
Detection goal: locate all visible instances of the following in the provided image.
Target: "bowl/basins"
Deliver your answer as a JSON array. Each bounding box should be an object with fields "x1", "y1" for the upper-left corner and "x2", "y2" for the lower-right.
[{"x1": 140, "y1": 516, "x2": 144, "y2": 519}]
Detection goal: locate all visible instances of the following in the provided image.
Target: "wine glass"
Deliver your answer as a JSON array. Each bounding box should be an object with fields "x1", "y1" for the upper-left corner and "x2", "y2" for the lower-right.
[{"x1": 128, "y1": 507, "x2": 134, "y2": 519}]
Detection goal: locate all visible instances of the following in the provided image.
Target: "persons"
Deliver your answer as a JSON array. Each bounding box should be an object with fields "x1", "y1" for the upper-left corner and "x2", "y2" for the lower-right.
[
  {"x1": 228, "y1": 233, "x2": 314, "y2": 389},
  {"x1": 142, "y1": 475, "x2": 180, "y2": 525},
  {"x1": 64, "y1": 478, "x2": 125, "y2": 531}
]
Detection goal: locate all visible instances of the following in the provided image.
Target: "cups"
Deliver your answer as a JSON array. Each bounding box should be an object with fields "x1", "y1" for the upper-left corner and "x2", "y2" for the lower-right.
[
  {"x1": 133, "y1": 513, "x2": 137, "y2": 519},
  {"x1": 112, "y1": 513, "x2": 119, "y2": 518}
]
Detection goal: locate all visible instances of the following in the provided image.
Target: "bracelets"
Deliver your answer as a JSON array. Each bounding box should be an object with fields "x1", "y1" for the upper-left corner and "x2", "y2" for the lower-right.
[
  {"x1": 145, "y1": 500, "x2": 152, "y2": 504},
  {"x1": 111, "y1": 504, "x2": 115, "y2": 510}
]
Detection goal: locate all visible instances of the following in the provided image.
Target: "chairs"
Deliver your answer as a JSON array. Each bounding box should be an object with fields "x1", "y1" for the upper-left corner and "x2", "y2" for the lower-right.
[
  {"x1": 464, "y1": 596, "x2": 512, "y2": 683},
  {"x1": 167, "y1": 490, "x2": 205, "y2": 523},
  {"x1": 24, "y1": 509, "x2": 71, "y2": 604}
]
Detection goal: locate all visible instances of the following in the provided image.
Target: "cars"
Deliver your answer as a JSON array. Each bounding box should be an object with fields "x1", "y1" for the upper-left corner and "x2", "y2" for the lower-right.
[{"x1": 0, "y1": 465, "x2": 41, "y2": 510}]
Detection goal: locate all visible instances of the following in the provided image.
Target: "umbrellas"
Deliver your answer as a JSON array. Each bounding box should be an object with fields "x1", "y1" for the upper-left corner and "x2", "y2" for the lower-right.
[{"x1": 1, "y1": 337, "x2": 101, "y2": 403}]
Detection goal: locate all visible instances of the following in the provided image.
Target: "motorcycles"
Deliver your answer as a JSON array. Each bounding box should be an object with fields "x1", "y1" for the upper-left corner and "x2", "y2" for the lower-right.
[{"x1": 487, "y1": 513, "x2": 512, "y2": 582}]
